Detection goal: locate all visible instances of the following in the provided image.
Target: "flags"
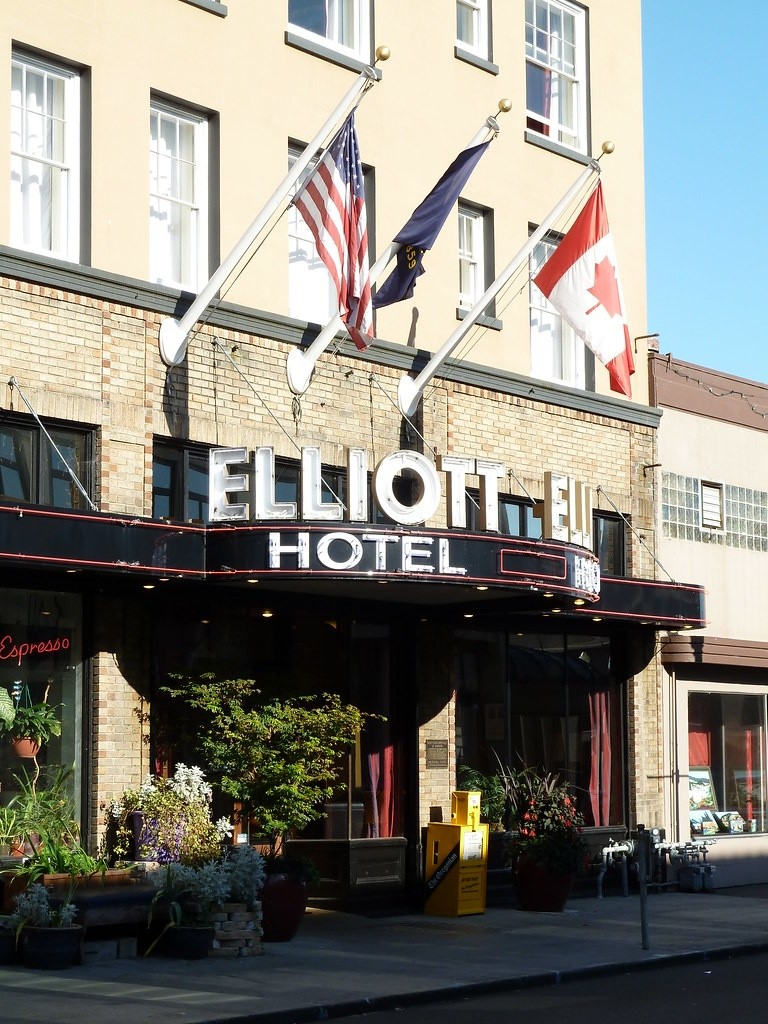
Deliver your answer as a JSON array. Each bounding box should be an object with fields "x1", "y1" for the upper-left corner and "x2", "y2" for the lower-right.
[
  {"x1": 372, "y1": 133, "x2": 498, "y2": 307},
  {"x1": 534, "y1": 181, "x2": 635, "y2": 399},
  {"x1": 290, "y1": 106, "x2": 374, "y2": 349}
]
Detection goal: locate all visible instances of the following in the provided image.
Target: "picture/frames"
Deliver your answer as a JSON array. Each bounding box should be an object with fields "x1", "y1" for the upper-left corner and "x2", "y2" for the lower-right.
[{"x1": 689, "y1": 766, "x2": 719, "y2": 813}]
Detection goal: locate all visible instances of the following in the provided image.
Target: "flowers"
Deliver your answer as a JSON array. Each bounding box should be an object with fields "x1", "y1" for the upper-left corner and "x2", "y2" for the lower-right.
[
  {"x1": 0, "y1": 892, "x2": 28, "y2": 929},
  {"x1": 489, "y1": 745, "x2": 593, "y2": 870},
  {"x1": 0, "y1": 680, "x2": 68, "y2": 747}
]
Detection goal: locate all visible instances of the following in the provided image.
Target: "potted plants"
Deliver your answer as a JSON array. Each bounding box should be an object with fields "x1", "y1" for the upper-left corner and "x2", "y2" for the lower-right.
[
  {"x1": 143, "y1": 857, "x2": 233, "y2": 960},
  {"x1": 159, "y1": 672, "x2": 390, "y2": 944},
  {"x1": 10, "y1": 883, "x2": 84, "y2": 971},
  {"x1": 0, "y1": 756, "x2": 80, "y2": 857}
]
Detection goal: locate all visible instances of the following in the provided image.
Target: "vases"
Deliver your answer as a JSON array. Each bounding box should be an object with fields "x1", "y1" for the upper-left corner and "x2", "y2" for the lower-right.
[
  {"x1": 0, "y1": 927, "x2": 22, "y2": 966},
  {"x1": 8, "y1": 734, "x2": 42, "y2": 758},
  {"x1": 510, "y1": 855, "x2": 576, "y2": 912}
]
